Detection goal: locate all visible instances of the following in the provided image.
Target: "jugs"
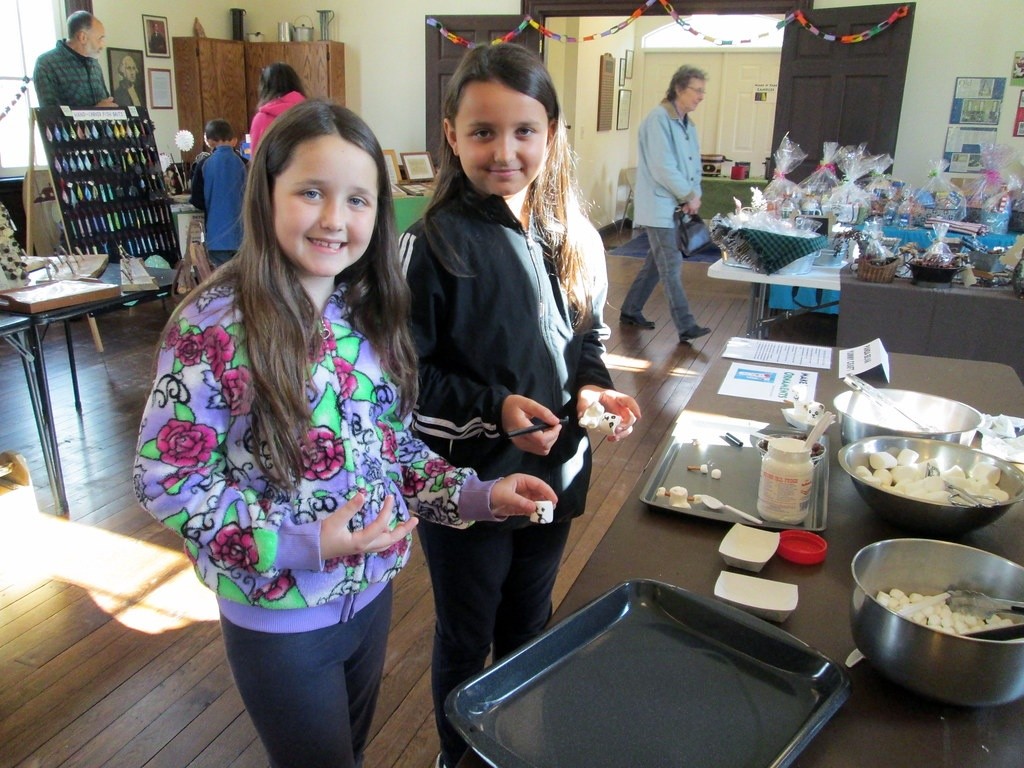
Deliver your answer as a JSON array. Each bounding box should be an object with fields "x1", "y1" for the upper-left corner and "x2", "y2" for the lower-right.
[{"x1": 278, "y1": 22, "x2": 291, "y2": 42}]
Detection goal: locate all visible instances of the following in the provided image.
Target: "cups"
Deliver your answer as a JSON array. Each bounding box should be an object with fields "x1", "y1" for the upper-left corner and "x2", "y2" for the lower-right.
[
  {"x1": 731, "y1": 166, "x2": 745, "y2": 179},
  {"x1": 735, "y1": 161, "x2": 751, "y2": 179}
]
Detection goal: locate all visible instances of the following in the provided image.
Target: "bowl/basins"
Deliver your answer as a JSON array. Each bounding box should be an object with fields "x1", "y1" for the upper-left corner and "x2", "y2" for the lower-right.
[
  {"x1": 248, "y1": 31, "x2": 264, "y2": 42},
  {"x1": 714, "y1": 570, "x2": 798, "y2": 622},
  {"x1": 758, "y1": 434, "x2": 826, "y2": 470},
  {"x1": 905, "y1": 262, "x2": 967, "y2": 288},
  {"x1": 719, "y1": 523, "x2": 780, "y2": 573},
  {"x1": 833, "y1": 388, "x2": 985, "y2": 446},
  {"x1": 851, "y1": 537, "x2": 1024, "y2": 706},
  {"x1": 838, "y1": 435, "x2": 1024, "y2": 532}
]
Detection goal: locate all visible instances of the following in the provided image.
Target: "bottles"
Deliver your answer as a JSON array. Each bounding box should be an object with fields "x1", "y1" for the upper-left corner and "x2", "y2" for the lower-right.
[
  {"x1": 230, "y1": 8, "x2": 246, "y2": 40},
  {"x1": 757, "y1": 438, "x2": 813, "y2": 524}
]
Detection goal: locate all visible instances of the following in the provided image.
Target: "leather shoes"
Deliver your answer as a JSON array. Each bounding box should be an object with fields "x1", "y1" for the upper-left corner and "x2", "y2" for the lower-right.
[
  {"x1": 619, "y1": 313, "x2": 655, "y2": 327},
  {"x1": 680, "y1": 325, "x2": 710, "y2": 342}
]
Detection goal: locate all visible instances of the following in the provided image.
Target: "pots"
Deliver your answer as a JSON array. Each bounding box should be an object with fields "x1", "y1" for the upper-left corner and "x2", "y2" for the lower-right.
[{"x1": 700, "y1": 155, "x2": 732, "y2": 176}]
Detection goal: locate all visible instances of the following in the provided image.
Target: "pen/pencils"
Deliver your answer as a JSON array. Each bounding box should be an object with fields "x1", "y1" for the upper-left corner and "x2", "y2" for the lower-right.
[{"x1": 505, "y1": 418, "x2": 567, "y2": 439}]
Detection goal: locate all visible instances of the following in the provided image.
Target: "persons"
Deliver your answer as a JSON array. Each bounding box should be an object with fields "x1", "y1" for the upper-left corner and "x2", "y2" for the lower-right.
[
  {"x1": 398, "y1": 40, "x2": 642, "y2": 767},
  {"x1": 619, "y1": 62, "x2": 712, "y2": 341},
  {"x1": 189, "y1": 119, "x2": 251, "y2": 266},
  {"x1": 132, "y1": 97, "x2": 558, "y2": 768},
  {"x1": 32, "y1": 11, "x2": 117, "y2": 107},
  {"x1": 250, "y1": 62, "x2": 308, "y2": 168}
]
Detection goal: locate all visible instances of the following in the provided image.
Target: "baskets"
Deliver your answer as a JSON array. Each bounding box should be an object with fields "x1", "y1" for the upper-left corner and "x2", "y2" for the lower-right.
[{"x1": 857, "y1": 252, "x2": 900, "y2": 283}]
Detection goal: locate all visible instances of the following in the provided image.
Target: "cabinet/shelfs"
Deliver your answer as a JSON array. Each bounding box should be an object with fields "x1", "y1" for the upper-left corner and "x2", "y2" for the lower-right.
[{"x1": 173, "y1": 34, "x2": 347, "y2": 161}]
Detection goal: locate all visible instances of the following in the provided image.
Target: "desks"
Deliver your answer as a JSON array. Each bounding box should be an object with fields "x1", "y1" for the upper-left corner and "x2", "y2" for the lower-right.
[
  {"x1": 1, "y1": 260, "x2": 205, "y2": 514},
  {"x1": 456, "y1": 178, "x2": 1024, "y2": 768}
]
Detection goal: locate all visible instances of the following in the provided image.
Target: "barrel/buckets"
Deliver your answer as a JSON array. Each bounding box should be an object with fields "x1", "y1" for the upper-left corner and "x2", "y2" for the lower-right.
[{"x1": 292, "y1": 15, "x2": 314, "y2": 41}]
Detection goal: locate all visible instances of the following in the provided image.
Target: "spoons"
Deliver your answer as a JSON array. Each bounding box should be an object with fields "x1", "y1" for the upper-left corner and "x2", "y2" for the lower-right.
[{"x1": 703, "y1": 495, "x2": 762, "y2": 525}]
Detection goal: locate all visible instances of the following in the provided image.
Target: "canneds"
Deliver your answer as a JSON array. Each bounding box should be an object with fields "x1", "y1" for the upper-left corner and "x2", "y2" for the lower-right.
[{"x1": 758, "y1": 437, "x2": 815, "y2": 525}]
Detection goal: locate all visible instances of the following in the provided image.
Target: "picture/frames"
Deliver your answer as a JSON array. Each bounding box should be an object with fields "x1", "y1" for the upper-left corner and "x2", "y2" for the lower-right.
[
  {"x1": 142, "y1": 14, "x2": 173, "y2": 57},
  {"x1": 626, "y1": 49, "x2": 634, "y2": 79},
  {"x1": 619, "y1": 57, "x2": 625, "y2": 87},
  {"x1": 149, "y1": 68, "x2": 174, "y2": 110},
  {"x1": 383, "y1": 149, "x2": 436, "y2": 196},
  {"x1": 616, "y1": 89, "x2": 630, "y2": 130},
  {"x1": 106, "y1": 47, "x2": 146, "y2": 111}
]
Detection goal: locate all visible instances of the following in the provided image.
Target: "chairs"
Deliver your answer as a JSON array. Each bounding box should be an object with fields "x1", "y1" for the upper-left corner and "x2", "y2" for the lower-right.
[{"x1": 620, "y1": 167, "x2": 642, "y2": 231}]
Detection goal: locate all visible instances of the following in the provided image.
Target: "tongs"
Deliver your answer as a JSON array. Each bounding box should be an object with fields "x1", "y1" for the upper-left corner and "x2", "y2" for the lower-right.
[
  {"x1": 947, "y1": 590, "x2": 1024, "y2": 640},
  {"x1": 925, "y1": 462, "x2": 1000, "y2": 508}
]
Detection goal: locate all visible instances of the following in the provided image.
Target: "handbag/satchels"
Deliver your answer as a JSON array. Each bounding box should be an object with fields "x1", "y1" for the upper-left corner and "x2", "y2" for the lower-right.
[{"x1": 674, "y1": 203, "x2": 712, "y2": 255}]
